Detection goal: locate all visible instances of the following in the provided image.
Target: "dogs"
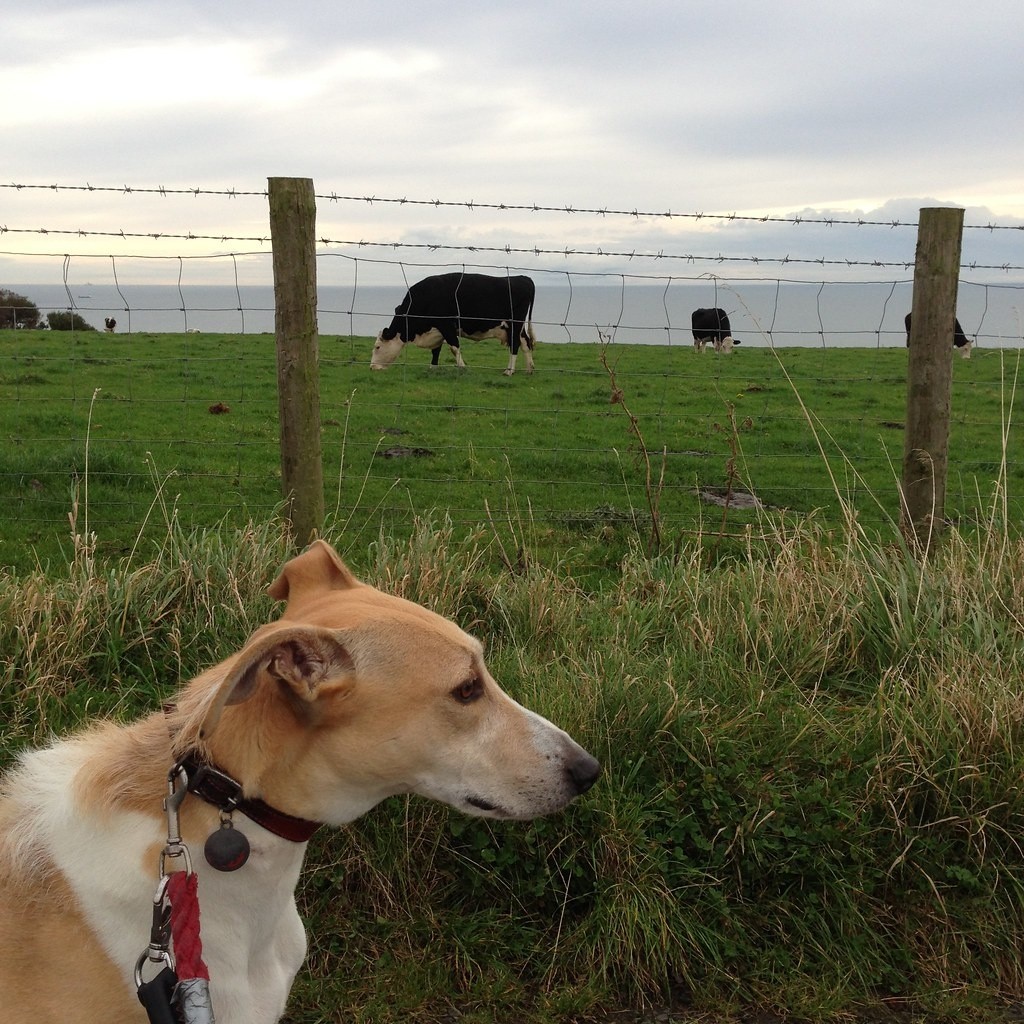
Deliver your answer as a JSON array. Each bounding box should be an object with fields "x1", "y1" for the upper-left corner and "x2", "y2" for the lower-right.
[{"x1": 0, "y1": 535, "x2": 607, "y2": 1024}]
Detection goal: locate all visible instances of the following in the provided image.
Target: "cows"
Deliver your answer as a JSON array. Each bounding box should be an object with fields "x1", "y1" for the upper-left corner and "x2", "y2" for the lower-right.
[
  {"x1": 904, "y1": 311, "x2": 975, "y2": 360},
  {"x1": 365, "y1": 270, "x2": 542, "y2": 379},
  {"x1": 691, "y1": 306, "x2": 742, "y2": 356}
]
